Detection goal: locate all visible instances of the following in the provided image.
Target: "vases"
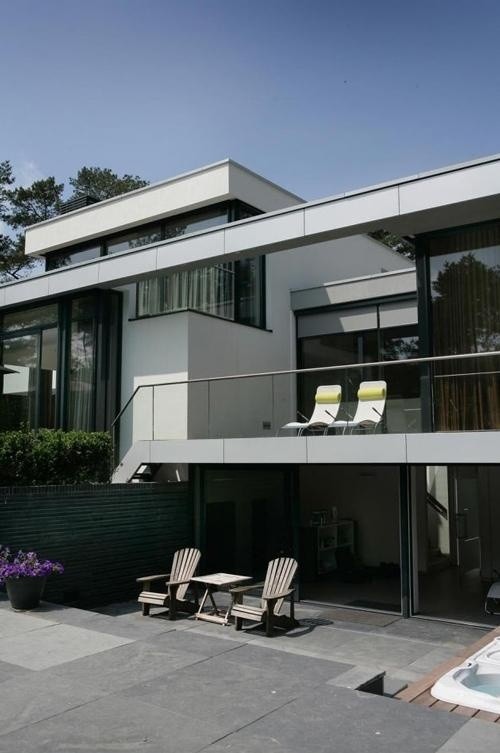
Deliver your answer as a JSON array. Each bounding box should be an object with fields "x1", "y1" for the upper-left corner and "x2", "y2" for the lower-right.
[{"x1": 6, "y1": 580, "x2": 42, "y2": 610}]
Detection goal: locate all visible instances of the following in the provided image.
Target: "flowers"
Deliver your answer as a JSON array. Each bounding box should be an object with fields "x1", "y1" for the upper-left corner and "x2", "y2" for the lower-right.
[{"x1": 0, "y1": 545, "x2": 64, "y2": 583}]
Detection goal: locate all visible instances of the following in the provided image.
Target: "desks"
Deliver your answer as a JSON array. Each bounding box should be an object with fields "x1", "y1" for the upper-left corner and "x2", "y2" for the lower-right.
[{"x1": 191, "y1": 572, "x2": 254, "y2": 627}]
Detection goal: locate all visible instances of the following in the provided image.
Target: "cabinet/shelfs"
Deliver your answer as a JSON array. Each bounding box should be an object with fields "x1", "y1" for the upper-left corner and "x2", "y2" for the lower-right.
[{"x1": 296, "y1": 518, "x2": 356, "y2": 577}]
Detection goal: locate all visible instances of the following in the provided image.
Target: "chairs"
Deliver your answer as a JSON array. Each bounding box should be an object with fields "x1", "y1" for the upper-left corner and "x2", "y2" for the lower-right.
[
  {"x1": 135, "y1": 547, "x2": 201, "y2": 621},
  {"x1": 325, "y1": 380, "x2": 388, "y2": 435},
  {"x1": 484, "y1": 581, "x2": 500, "y2": 615},
  {"x1": 229, "y1": 556, "x2": 302, "y2": 638},
  {"x1": 277, "y1": 384, "x2": 342, "y2": 436}
]
